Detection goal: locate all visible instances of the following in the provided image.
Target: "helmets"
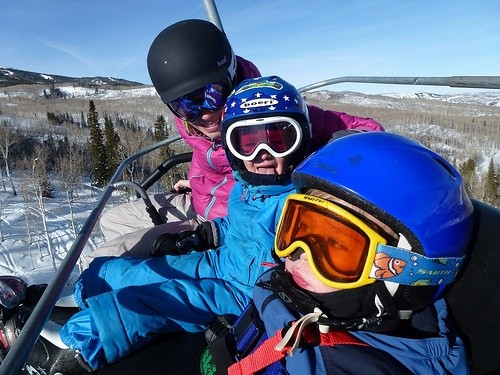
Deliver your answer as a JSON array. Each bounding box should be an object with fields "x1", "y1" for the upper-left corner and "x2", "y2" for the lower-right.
[
  {"x1": 144, "y1": 19, "x2": 238, "y2": 103},
  {"x1": 218, "y1": 76, "x2": 314, "y2": 186},
  {"x1": 290, "y1": 130, "x2": 476, "y2": 330}
]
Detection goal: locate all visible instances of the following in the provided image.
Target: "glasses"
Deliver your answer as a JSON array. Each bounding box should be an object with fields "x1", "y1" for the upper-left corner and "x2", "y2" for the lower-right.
[
  {"x1": 274, "y1": 192, "x2": 464, "y2": 291},
  {"x1": 226, "y1": 116, "x2": 301, "y2": 161},
  {"x1": 167, "y1": 78, "x2": 235, "y2": 121}
]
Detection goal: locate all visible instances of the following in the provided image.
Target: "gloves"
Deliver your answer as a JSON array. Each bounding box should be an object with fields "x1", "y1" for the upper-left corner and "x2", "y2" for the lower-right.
[{"x1": 146, "y1": 220, "x2": 218, "y2": 256}]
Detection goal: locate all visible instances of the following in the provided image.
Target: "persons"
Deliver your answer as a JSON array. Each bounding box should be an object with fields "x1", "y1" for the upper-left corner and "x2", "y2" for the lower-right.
[
  {"x1": 190, "y1": 131, "x2": 475, "y2": 375},
  {"x1": 81, "y1": 19, "x2": 383, "y2": 270},
  {"x1": 40, "y1": 75, "x2": 317, "y2": 371}
]
